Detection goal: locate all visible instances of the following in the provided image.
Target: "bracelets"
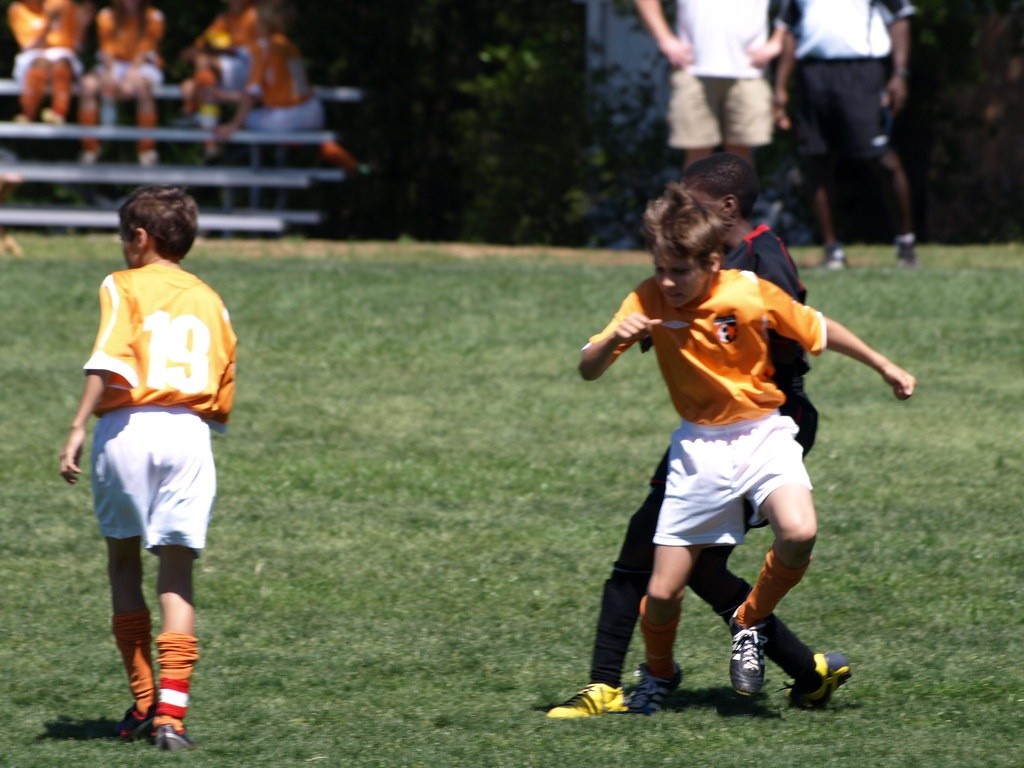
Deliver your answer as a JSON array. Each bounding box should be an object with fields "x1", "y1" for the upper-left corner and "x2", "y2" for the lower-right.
[{"x1": 892, "y1": 67, "x2": 909, "y2": 77}]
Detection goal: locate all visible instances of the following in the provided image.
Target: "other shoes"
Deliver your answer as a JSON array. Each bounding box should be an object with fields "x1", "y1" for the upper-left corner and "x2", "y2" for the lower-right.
[
  {"x1": 823, "y1": 244, "x2": 849, "y2": 273},
  {"x1": 898, "y1": 240, "x2": 918, "y2": 268}
]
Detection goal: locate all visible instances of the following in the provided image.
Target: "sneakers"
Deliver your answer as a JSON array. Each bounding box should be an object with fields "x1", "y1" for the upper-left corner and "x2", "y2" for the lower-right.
[
  {"x1": 150, "y1": 724, "x2": 193, "y2": 751},
  {"x1": 728, "y1": 603, "x2": 767, "y2": 697},
  {"x1": 774, "y1": 653, "x2": 852, "y2": 709},
  {"x1": 545, "y1": 683, "x2": 630, "y2": 720},
  {"x1": 119, "y1": 702, "x2": 156, "y2": 741},
  {"x1": 629, "y1": 660, "x2": 683, "y2": 715}
]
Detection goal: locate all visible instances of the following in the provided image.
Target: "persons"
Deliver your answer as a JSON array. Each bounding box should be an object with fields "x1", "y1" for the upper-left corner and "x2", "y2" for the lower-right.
[
  {"x1": 579, "y1": 182, "x2": 916, "y2": 712},
  {"x1": 635, "y1": 0, "x2": 916, "y2": 274},
  {"x1": 7, "y1": 0, "x2": 167, "y2": 167},
  {"x1": 545, "y1": 153, "x2": 852, "y2": 718},
  {"x1": 180, "y1": 0, "x2": 368, "y2": 174},
  {"x1": 59, "y1": 186, "x2": 238, "y2": 748}
]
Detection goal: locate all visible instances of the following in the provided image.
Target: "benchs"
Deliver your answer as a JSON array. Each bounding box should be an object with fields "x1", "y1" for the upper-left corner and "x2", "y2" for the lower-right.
[{"x1": 0, "y1": 78, "x2": 360, "y2": 239}]
